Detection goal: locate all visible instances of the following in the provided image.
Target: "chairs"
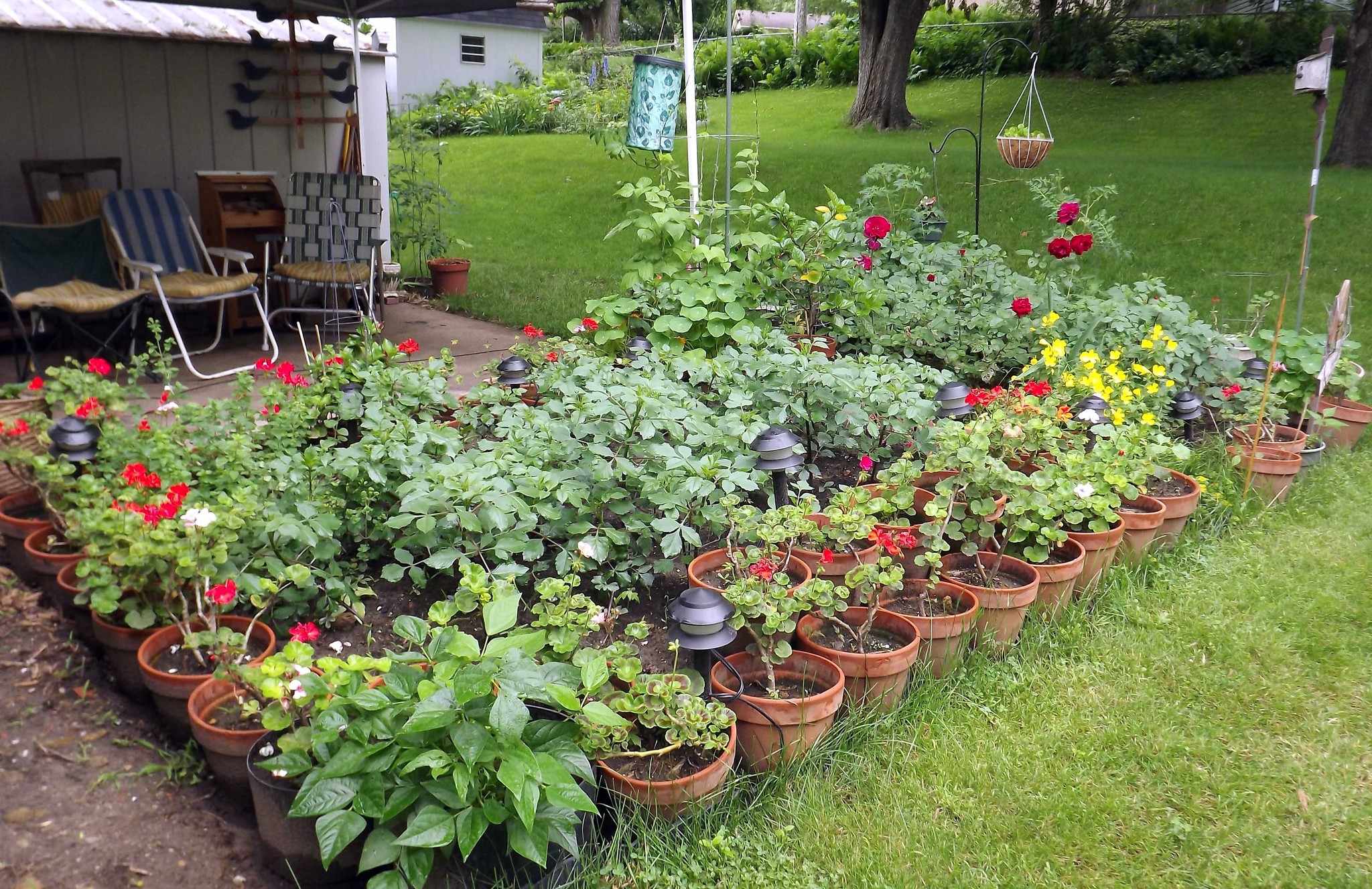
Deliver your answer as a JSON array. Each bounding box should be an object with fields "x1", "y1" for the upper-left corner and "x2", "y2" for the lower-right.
[
  {"x1": 0, "y1": 218, "x2": 152, "y2": 386},
  {"x1": 274, "y1": 170, "x2": 387, "y2": 335},
  {"x1": 21, "y1": 155, "x2": 122, "y2": 223},
  {"x1": 102, "y1": 187, "x2": 280, "y2": 379}
]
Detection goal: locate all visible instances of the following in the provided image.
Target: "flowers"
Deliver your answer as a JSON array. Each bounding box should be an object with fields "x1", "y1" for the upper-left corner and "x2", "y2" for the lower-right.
[{"x1": 0, "y1": 196, "x2": 1372, "y2": 732}]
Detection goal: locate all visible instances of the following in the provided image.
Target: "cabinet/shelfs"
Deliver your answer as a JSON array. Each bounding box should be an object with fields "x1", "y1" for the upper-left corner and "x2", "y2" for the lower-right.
[{"x1": 197, "y1": 171, "x2": 284, "y2": 269}]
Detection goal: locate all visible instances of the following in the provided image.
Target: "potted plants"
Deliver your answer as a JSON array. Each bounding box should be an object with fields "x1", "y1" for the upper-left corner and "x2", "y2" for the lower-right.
[
  {"x1": 387, "y1": 91, "x2": 471, "y2": 296},
  {"x1": 995, "y1": 123, "x2": 1055, "y2": 170},
  {"x1": 254, "y1": 613, "x2": 601, "y2": 889}
]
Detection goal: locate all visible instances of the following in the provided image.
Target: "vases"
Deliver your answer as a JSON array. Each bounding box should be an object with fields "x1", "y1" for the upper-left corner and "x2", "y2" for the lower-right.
[
  {"x1": 0, "y1": 484, "x2": 356, "y2": 864},
  {"x1": 909, "y1": 219, "x2": 949, "y2": 242},
  {"x1": 601, "y1": 357, "x2": 1372, "y2": 844}
]
